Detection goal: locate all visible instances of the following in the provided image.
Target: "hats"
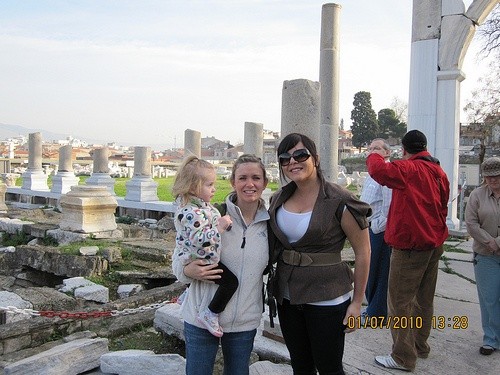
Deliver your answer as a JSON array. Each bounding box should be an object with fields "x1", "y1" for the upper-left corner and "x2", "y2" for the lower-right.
[
  {"x1": 480, "y1": 157, "x2": 500, "y2": 177},
  {"x1": 402, "y1": 130, "x2": 427, "y2": 152}
]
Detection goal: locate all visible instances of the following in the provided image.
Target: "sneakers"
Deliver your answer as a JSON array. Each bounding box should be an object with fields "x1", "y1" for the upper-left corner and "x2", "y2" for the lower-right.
[
  {"x1": 195, "y1": 308, "x2": 223, "y2": 337},
  {"x1": 357, "y1": 311, "x2": 388, "y2": 327},
  {"x1": 375, "y1": 356, "x2": 411, "y2": 371},
  {"x1": 176, "y1": 287, "x2": 189, "y2": 305}
]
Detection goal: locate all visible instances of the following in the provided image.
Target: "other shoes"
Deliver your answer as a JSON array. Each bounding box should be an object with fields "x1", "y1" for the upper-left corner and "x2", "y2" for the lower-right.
[{"x1": 479, "y1": 345, "x2": 496, "y2": 356}]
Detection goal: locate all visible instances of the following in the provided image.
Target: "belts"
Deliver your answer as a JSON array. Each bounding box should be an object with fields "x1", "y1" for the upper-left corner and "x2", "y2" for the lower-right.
[{"x1": 278, "y1": 250, "x2": 341, "y2": 266}]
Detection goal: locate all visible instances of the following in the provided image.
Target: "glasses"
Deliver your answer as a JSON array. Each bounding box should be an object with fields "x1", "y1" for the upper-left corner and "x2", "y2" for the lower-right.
[
  {"x1": 368, "y1": 147, "x2": 387, "y2": 153},
  {"x1": 278, "y1": 149, "x2": 312, "y2": 166}
]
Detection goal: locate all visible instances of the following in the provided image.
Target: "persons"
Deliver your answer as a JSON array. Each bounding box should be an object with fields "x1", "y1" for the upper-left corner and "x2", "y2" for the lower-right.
[
  {"x1": 360, "y1": 138, "x2": 393, "y2": 326},
  {"x1": 366, "y1": 130, "x2": 449, "y2": 371},
  {"x1": 171, "y1": 153, "x2": 239, "y2": 337},
  {"x1": 264, "y1": 133, "x2": 373, "y2": 375},
  {"x1": 171, "y1": 154, "x2": 270, "y2": 375},
  {"x1": 464, "y1": 157, "x2": 500, "y2": 356}
]
